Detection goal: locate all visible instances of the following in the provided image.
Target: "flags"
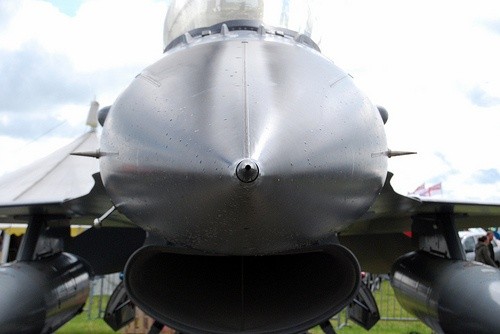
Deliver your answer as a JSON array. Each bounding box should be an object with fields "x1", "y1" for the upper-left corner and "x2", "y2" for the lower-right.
[
  {"x1": 407, "y1": 183, "x2": 426, "y2": 196},
  {"x1": 419, "y1": 182, "x2": 443, "y2": 198}
]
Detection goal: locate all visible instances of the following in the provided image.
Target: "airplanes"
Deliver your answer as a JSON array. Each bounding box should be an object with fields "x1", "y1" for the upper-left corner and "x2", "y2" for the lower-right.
[{"x1": 0, "y1": 0, "x2": 497, "y2": 334}]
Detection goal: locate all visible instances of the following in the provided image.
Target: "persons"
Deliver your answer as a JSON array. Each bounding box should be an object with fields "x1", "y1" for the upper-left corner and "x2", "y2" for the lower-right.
[
  {"x1": 486, "y1": 231, "x2": 498, "y2": 268},
  {"x1": 474, "y1": 236, "x2": 498, "y2": 269}
]
